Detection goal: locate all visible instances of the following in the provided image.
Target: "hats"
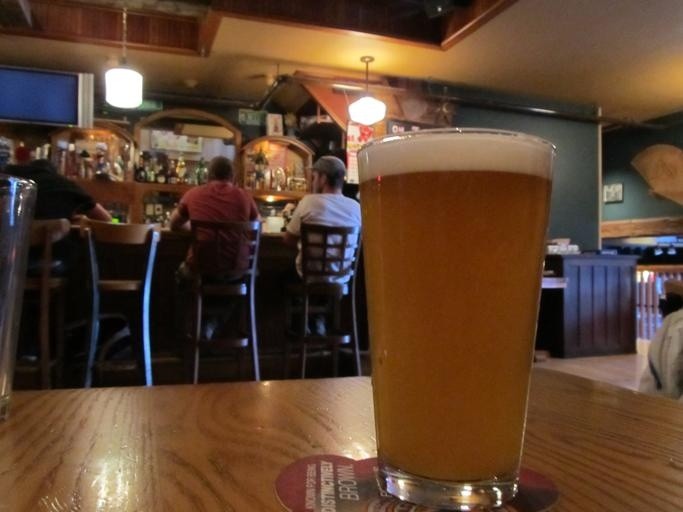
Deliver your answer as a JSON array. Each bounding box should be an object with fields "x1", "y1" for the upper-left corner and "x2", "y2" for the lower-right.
[{"x1": 305, "y1": 156, "x2": 346, "y2": 177}]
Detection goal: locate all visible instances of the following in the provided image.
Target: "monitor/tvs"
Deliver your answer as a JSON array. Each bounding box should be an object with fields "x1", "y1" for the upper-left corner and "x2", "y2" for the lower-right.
[{"x1": 1, "y1": 64, "x2": 81, "y2": 129}]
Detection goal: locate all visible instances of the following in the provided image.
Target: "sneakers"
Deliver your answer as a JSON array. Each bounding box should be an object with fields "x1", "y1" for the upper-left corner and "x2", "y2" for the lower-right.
[
  {"x1": 223, "y1": 337, "x2": 249, "y2": 348},
  {"x1": 313, "y1": 318, "x2": 326, "y2": 337},
  {"x1": 186, "y1": 327, "x2": 213, "y2": 344}
]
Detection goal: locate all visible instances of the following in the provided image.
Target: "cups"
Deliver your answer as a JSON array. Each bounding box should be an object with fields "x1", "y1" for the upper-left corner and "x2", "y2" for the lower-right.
[
  {"x1": 0, "y1": 172, "x2": 40, "y2": 423},
  {"x1": 356, "y1": 125, "x2": 558, "y2": 511}
]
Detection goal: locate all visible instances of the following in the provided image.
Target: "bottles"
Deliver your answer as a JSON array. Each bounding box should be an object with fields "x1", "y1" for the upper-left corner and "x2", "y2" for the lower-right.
[{"x1": 13, "y1": 141, "x2": 307, "y2": 232}]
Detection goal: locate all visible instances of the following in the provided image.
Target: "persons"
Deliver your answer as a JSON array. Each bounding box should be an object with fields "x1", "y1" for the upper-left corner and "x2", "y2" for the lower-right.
[
  {"x1": 280, "y1": 154, "x2": 361, "y2": 341},
  {"x1": 0, "y1": 155, "x2": 115, "y2": 361},
  {"x1": 167, "y1": 155, "x2": 259, "y2": 338},
  {"x1": 631, "y1": 307, "x2": 682, "y2": 404}
]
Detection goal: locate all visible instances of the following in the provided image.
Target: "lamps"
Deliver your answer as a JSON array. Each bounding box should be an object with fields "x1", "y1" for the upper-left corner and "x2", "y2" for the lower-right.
[
  {"x1": 344, "y1": 56, "x2": 387, "y2": 127},
  {"x1": 101, "y1": 1, "x2": 142, "y2": 112}
]
